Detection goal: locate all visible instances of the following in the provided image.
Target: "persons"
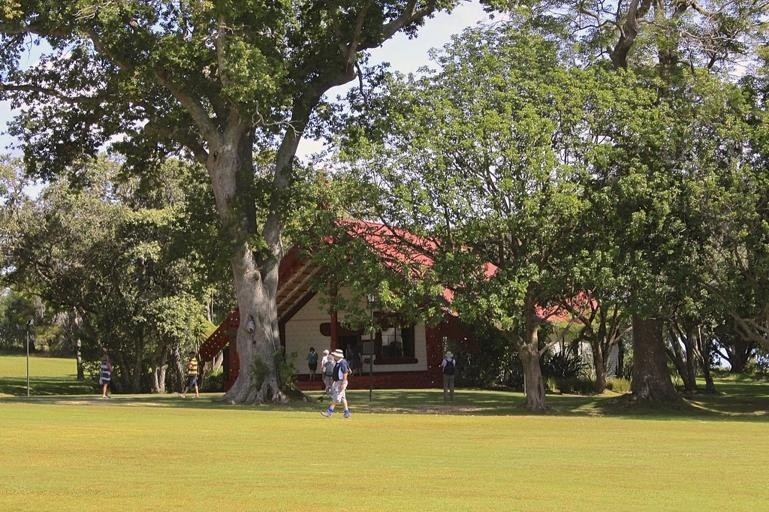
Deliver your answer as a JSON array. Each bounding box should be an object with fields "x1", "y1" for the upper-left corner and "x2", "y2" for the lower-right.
[
  {"x1": 304, "y1": 347, "x2": 362, "y2": 418},
  {"x1": 438, "y1": 351, "x2": 456, "y2": 401},
  {"x1": 98, "y1": 354, "x2": 114, "y2": 399},
  {"x1": 179, "y1": 351, "x2": 199, "y2": 399}
]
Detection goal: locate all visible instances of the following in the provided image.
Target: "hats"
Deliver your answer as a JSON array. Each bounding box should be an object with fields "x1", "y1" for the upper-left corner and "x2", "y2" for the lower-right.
[
  {"x1": 332, "y1": 349, "x2": 344, "y2": 358},
  {"x1": 445, "y1": 351, "x2": 453, "y2": 357}
]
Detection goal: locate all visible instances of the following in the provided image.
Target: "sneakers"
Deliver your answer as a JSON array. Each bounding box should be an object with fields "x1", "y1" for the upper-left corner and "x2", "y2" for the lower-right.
[{"x1": 321, "y1": 409, "x2": 352, "y2": 418}]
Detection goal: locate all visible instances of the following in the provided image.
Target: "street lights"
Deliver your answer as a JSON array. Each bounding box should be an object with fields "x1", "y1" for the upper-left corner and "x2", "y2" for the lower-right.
[
  {"x1": 368, "y1": 293, "x2": 375, "y2": 402},
  {"x1": 26, "y1": 319, "x2": 36, "y2": 396}
]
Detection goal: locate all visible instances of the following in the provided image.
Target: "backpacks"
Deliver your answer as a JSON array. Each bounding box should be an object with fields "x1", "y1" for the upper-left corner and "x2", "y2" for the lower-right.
[{"x1": 443, "y1": 359, "x2": 456, "y2": 375}]
